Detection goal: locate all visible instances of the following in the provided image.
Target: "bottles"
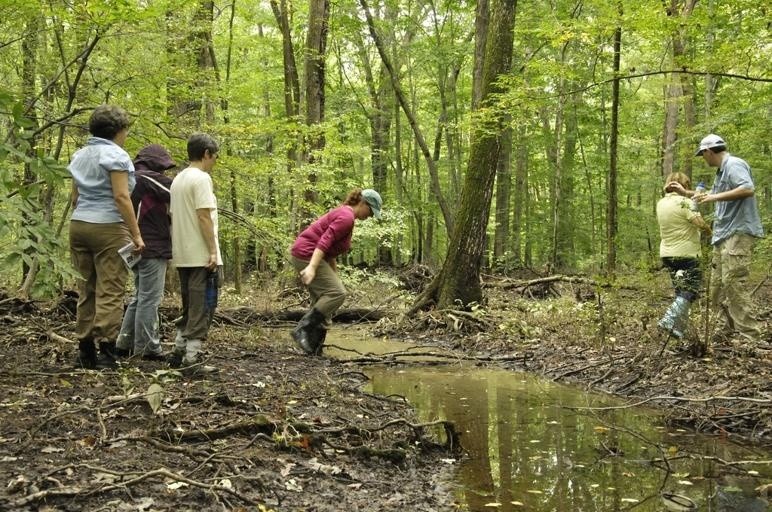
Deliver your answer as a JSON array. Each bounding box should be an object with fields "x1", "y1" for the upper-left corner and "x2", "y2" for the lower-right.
[
  {"x1": 690, "y1": 181, "x2": 704, "y2": 211},
  {"x1": 206, "y1": 271, "x2": 218, "y2": 307}
]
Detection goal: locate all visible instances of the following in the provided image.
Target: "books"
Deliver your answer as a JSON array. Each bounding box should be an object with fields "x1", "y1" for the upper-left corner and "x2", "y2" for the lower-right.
[{"x1": 118, "y1": 241, "x2": 143, "y2": 270}]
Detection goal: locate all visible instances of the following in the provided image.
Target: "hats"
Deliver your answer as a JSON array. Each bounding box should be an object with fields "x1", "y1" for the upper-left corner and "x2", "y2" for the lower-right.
[
  {"x1": 363, "y1": 189, "x2": 383, "y2": 221},
  {"x1": 694, "y1": 135, "x2": 725, "y2": 156}
]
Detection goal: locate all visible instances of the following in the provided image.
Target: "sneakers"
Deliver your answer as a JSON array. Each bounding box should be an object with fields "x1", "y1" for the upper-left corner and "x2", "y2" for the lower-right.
[
  {"x1": 140, "y1": 352, "x2": 170, "y2": 360},
  {"x1": 116, "y1": 348, "x2": 132, "y2": 356},
  {"x1": 185, "y1": 364, "x2": 219, "y2": 376},
  {"x1": 166, "y1": 356, "x2": 180, "y2": 367}
]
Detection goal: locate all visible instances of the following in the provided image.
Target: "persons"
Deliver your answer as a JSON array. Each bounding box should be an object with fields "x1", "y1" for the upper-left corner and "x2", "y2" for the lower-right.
[
  {"x1": 660, "y1": 131, "x2": 765, "y2": 351},
  {"x1": 112, "y1": 143, "x2": 177, "y2": 364},
  {"x1": 285, "y1": 187, "x2": 383, "y2": 355},
  {"x1": 64, "y1": 103, "x2": 146, "y2": 370},
  {"x1": 166, "y1": 132, "x2": 227, "y2": 377},
  {"x1": 655, "y1": 171, "x2": 711, "y2": 342}
]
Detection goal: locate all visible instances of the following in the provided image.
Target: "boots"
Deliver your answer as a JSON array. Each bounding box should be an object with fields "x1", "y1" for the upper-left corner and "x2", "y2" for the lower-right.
[
  {"x1": 75, "y1": 340, "x2": 97, "y2": 369},
  {"x1": 659, "y1": 297, "x2": 691, "y2": 338},
  {"x1": 97, "y1": 343, "x2": 118, "y2": 371},
  {"x1": 291, "y1": 309, "x2": 326, "y2": 355}
]
